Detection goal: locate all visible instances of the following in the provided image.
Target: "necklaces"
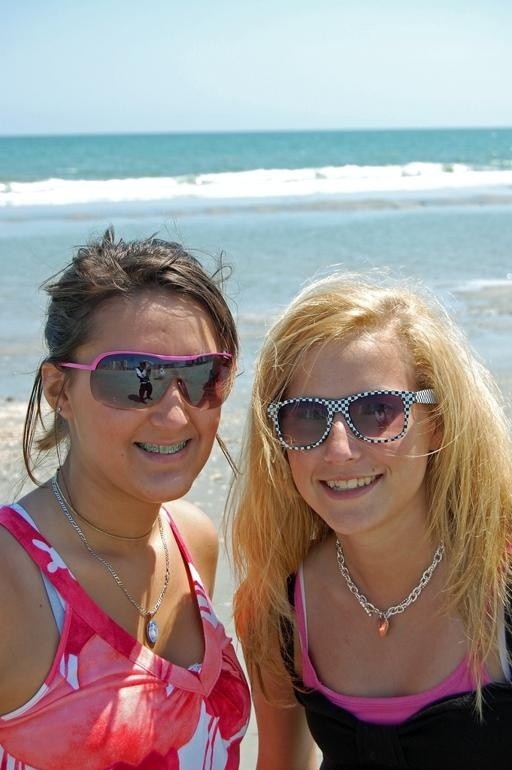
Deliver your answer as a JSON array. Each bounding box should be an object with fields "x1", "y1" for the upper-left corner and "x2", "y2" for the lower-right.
[
  {"x1": 333, "y1": 529, "x2": 449, "y2": 637},
  {"x1": 54, "y1": 478, "x2": 162, "y2": 542},
  {"x1": 49, "y1": 464, "x2": 173, "y2": 652}
]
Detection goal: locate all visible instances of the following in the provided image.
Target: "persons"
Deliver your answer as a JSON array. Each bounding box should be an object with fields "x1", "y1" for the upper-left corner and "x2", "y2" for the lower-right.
[
  {"x1": 134, "y1": 357, "x2": 156, "y2": 405},
  {"x1": 372, "y1": 401, "x2": 388, "y2": 432},
  {"x1": 222, "y1": 264, "x2": 512, "y2": 767},
  {"x1": 2, "y1": 218, "x2": 253, "y2": 770}
]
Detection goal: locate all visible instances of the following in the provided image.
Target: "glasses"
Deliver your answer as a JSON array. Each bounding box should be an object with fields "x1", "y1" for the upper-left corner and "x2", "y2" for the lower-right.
[
  {"x1": 51, "y1": 351, "x2": 237, "y2": 413},
  {"x1": 264, "y1": 387, "x2": 439, "y2": 453}
]
